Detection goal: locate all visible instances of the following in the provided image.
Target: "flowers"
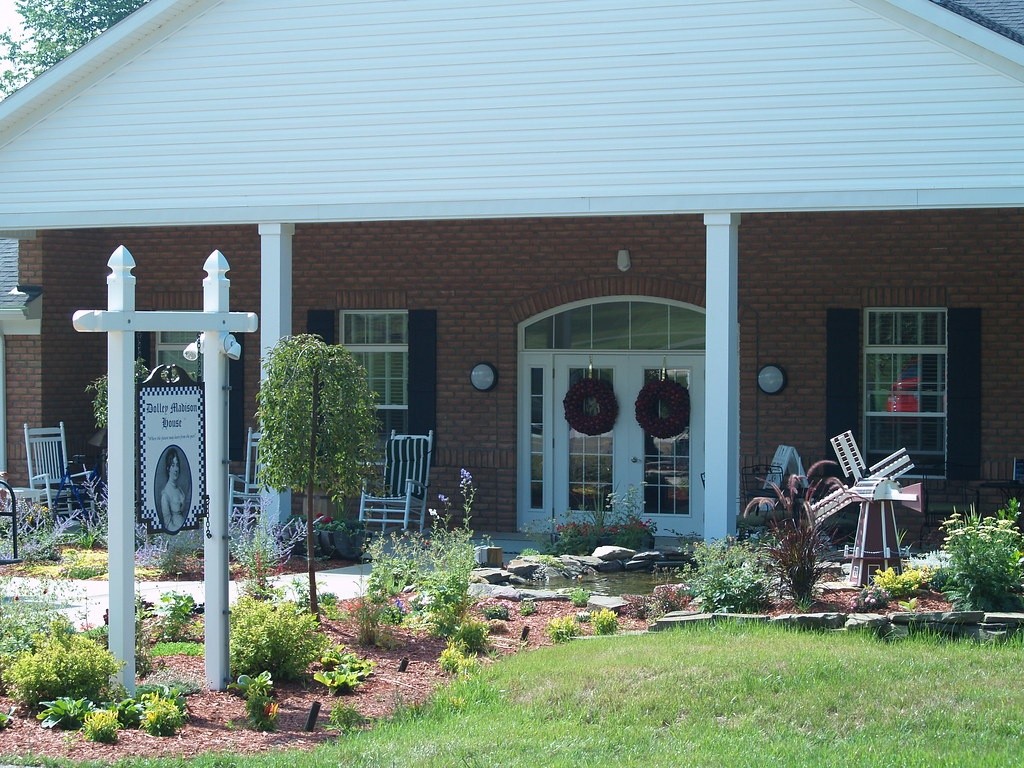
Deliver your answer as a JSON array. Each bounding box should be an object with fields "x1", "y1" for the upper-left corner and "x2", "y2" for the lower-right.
[
  {"x1": 280, "y1": 511, "x2": 346, "y2": 530},
  {"x1": 555, "y1": 480, "x2": 657, "y2": 538}
]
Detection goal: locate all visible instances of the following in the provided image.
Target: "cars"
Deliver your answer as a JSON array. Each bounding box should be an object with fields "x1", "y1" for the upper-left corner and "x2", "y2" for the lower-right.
[{"x1": 887, "y1": 357, "x2": 919, "y2": 411}]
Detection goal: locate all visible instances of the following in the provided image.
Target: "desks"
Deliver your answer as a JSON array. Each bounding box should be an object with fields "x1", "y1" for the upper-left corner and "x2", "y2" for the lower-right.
[
  {"x1": 744, "y1": 486, "x2": 819, "y2": 522},
  {"x1": 982, "y1": 482, "x2": 1024, "y2": 508}
]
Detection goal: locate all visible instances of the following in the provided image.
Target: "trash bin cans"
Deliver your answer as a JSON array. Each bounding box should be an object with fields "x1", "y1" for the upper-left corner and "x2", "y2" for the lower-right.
[{"x1": 303, "y1": 488, "x2": 346, "y2": 519}]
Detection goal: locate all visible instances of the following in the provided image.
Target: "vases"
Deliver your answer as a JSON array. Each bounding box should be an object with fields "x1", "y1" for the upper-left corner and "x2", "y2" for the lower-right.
[
  {"x1": 551, "y1": 523, "x2": 655, "y2": 556},
  {"x1": 271, "y1": 524, "x2": 372, "y2": 561}
]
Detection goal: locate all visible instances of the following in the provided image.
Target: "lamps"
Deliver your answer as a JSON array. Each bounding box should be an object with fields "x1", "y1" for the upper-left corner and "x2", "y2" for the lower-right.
[
  {"x1": 756, "y1": 364, "x2": 787, "y2": 393},
  {"x1": 471, "y1": 362, "x2": 499, "y2": 391},
  {"x1": 183, "y1": 333, "x2": 205, "y2": 361},
  {"x1": 225, "y1": 335, "x2": 241, "y2": 361}
]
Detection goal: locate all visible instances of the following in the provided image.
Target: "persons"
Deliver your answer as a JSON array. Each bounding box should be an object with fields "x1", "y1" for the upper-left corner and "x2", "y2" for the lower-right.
[{"x1": 160, "y1": 448, "x2": 185, "y2": 531}]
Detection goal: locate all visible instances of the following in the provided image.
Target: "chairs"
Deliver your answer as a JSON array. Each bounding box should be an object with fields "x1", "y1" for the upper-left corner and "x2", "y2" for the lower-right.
[
  {"x1": 229, "y1": 426, "x2": 266, "y2": 526},
  {"x1": 920, "y1": 468, "x2": 979, "y2": 549},
  {"x1": 355, "y1": 429, "x2": 433, "y2": 539},
  {"x1": 822, "y1": 512, "x2": 858, "y2": 547},
  {"x1": 24, "y1": 422, "x2": 96, "y2": 529},
  {"x1": 742, "y1": 465, "x2": 791, "y2": 527},
  {"x1": 1, "y1": 486, "x2": 40, "y2": 503},
  {"x1": 701, "y1": 472, "x2": 766, "y2": 536}
]
